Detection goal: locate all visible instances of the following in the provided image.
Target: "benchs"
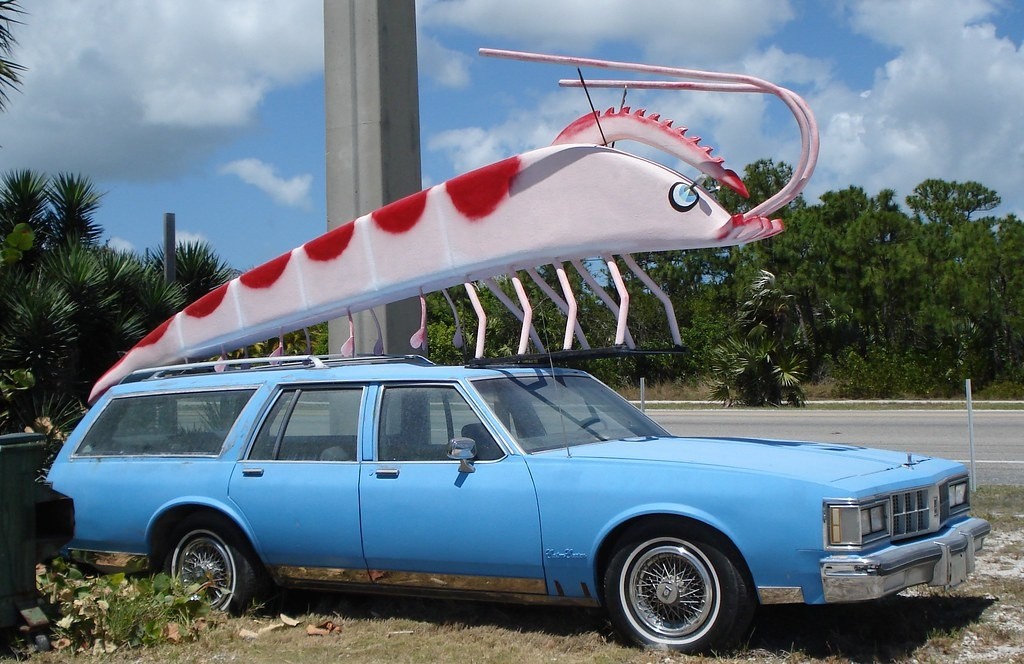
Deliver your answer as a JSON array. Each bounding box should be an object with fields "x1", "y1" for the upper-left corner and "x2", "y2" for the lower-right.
[{"x1": 160, "y1": 430, "x2": 231, "y2": 455}]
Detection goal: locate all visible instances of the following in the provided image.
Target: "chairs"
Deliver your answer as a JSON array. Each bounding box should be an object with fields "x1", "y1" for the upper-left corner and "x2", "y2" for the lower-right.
[
  {"x1": 320, "y1": 443, "x2": 357, "y2": 461},
  {"x1": 462, "y1": 423, "x2": 498, "y2": 461}
]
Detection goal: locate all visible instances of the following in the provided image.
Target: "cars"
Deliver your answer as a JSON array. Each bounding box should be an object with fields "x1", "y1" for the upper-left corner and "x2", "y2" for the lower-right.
[{"x1": 42, "y1": 352, "x2": 991, "y2": 658}]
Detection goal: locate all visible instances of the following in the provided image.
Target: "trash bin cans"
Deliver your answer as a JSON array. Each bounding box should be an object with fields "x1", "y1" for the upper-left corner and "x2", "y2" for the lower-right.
[{"x1": 0, "y1": 432, "x2": 47, "y2": 630}]
